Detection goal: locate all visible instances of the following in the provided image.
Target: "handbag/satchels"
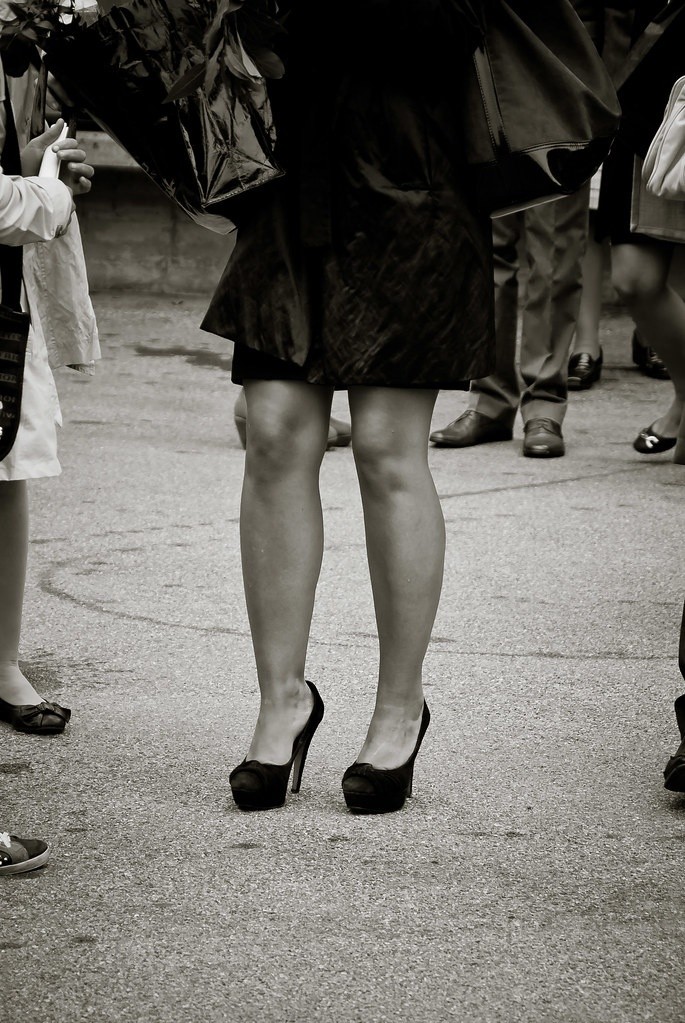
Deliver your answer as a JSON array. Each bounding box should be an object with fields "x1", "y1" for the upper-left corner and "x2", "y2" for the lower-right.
[{"x1": 42, "y1": 0, "x2": 284, "y2": 237}]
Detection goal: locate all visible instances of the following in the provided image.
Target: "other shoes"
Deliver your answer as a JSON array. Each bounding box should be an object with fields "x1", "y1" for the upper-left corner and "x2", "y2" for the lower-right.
[
  {"x1": 631, "y1": 332, "x2": 672, "y2": 379},
  {"x1": 0, "y1": 698, "x2": 71, "y2": 735},
  {"x1": 634, "y1": 419, "x2": 677, "y2": 454},
  {"x1": 523, "y1": 418, "x2": 565, "y2": 457},
  {"x1": 566, "y1": 345, "x2": 603, "y2": 390},
  {"x1": 0, "y1": 830, "x2": 49, "y2": 875},
  {"x1": 430, "y1": 410, "x2": 512, "y2": 446}
]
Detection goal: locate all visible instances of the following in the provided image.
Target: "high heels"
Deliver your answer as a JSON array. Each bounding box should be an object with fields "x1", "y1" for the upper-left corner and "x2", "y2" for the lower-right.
[
  {"x1": 234, "y1": 410, "x2": 351, "y2": 450},
  {"x1": 343, "y1": 699, "x2": 430, "y2": 813},
  {"x1": 230, "y1": 680, "x2": 324, "y2": 811}
]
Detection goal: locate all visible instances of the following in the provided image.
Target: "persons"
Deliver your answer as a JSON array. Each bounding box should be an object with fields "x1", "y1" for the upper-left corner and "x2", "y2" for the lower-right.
[
  {"x1": 0, "y1": 8, "x2": 97, "y2": 882},
  {"x1": 0, "y1": 16, "x2": 101, "y2": 736},
  {"x1": 228, "y1": 381, "x2": 354, "y2": 456},
  {"x1": 563, "y1": 126, "x2": 677, "y2": 394},
  {"x1": 200, "y1": 1, "x2": 534, "y2": 818},
  {"x1": 426, "y1": 175, "x2": 607, "y2": 460},
  {"x1": 596, "y1": 1, "x2": 685, "y2": 465}
]
